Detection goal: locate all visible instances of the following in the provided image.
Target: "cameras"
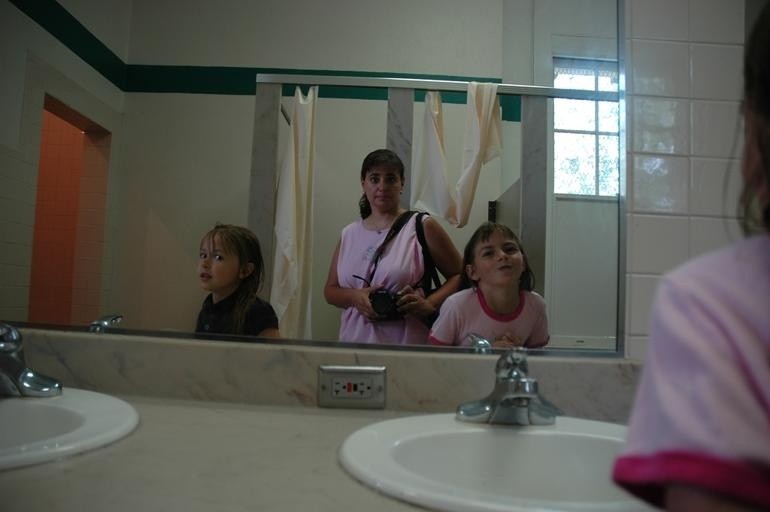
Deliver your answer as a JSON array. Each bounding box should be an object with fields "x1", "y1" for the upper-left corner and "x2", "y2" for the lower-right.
[{"x1": 368, "y1": 287, "x2": 407, "y2": 322}]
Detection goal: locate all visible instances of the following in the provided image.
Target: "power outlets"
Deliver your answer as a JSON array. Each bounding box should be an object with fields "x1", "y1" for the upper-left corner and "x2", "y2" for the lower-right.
[{"x1": 316, "y1": 363, "x2": 390, "y2": 413}]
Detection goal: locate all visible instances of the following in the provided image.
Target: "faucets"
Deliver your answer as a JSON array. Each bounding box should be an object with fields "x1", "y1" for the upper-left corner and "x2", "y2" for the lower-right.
[
  {"x1": 455, "y1": 349, "x2": 578, "y2": 427},
  {"x1": 465, "y1": 331, "x2": 494, "y2": 353},
  {"x1": 85, "y1": 313, "x2": 122, "y2": 333},
  {"x1": 0, "y1": 321, "x2": 63, "y2": 398}
]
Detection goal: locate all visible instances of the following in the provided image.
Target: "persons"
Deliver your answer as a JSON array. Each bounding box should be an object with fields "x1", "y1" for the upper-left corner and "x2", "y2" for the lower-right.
[
  {"x1": 323, "y1": 149, "x2": 463, "y2": 346},
  {"x1": 612, "y1": 2, "x2": 769, "y2": 512},
  {"x1": 427, "y1": 220, "x2": 549, "y2": 351},
  {"x1": 195, "y1": 223, "x2": 285, "y2": 339}
]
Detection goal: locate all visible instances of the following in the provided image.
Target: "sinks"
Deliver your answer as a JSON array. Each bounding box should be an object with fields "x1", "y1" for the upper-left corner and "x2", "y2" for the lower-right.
[
  {"x1": 340, "y1": 411, "x2": 661, "y2": 510},
  {"x1": 1, "y1": 385, "x2": 138, "y2": 471}
]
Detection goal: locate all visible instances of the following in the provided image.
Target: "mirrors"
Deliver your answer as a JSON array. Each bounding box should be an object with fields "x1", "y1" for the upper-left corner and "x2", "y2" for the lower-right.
[{"x1": 0, "y1": 0, "x2": 639, "y2": 364}]
